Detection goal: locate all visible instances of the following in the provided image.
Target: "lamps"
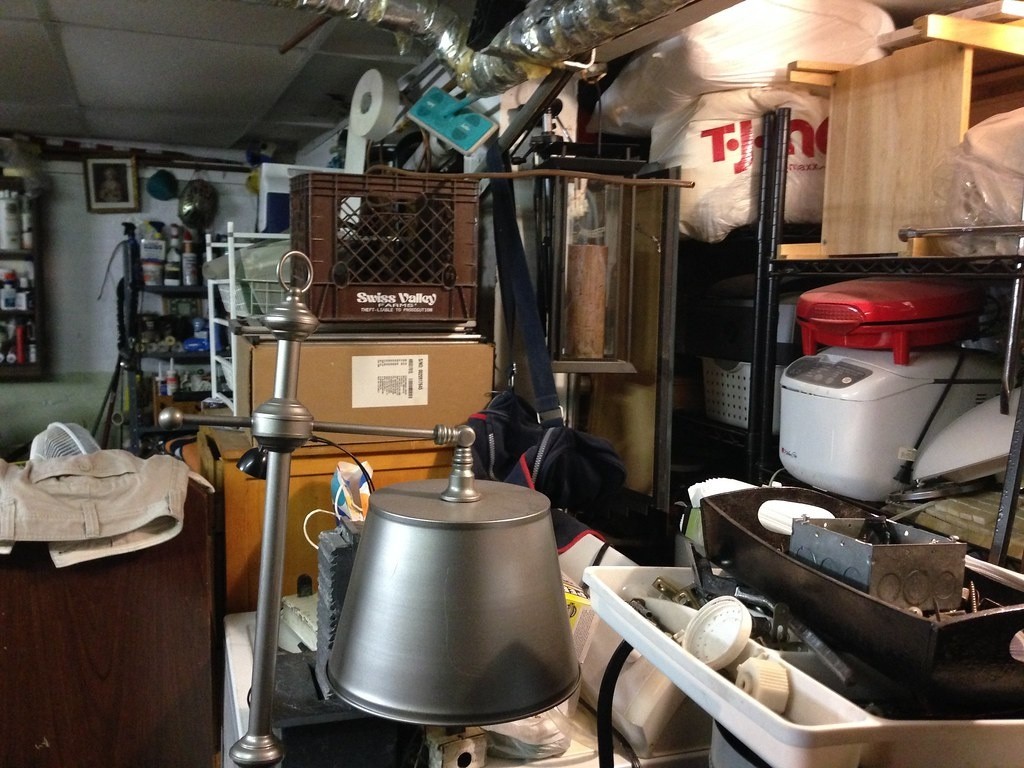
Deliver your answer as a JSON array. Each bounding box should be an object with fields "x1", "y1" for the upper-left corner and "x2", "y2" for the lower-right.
[{"x1": 160, "y1": 252, "x2": 583, "y2": 767}]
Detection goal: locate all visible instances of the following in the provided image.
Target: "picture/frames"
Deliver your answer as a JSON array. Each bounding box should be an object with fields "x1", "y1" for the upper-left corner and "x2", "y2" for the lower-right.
[{"x1": 82, "y1": 151, "x2": 141, "y2": 213}]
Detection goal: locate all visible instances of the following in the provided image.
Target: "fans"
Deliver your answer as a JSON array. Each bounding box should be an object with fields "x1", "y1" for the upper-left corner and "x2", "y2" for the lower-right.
[{"x1": 30, "y1": 422, "x2": 99, "y2": 463}]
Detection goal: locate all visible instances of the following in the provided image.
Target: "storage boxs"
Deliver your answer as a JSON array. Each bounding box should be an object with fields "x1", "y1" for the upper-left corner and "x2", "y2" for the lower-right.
[
  {"x1": 700, "y1": 356, "x2": 789, "y2": 432},
  {"x1": 289, "y1": 171, "x2": 482, "y2": 321},
  {"x1": 700, "y1": 487, "x2": 1023, "y2": 717},
  {"x1": 196, "y1": 424, "x2": 458, "y2": 613},
  {"x1": 246, "y1": 340, "x2": 496, "y2": 449},
  {"x1": 584, "y1": 563, "x2": 1024, "y2": 767}
]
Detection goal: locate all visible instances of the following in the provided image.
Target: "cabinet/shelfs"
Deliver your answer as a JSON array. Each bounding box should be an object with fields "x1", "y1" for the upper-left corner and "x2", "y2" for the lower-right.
[
  {"x1": 124, "y1": 237, "x2": 229, "y2": 459},
  {"x1": 669, "y1": 110, "x2": 776, "y2": 510},
  {"x1": 1, "y1": 480, "x2": 215, "y2": 767},
  {"x1": 0, "y1": 172, "x2": 46, "y2": 380},
  {"x1": 758, "y1": 103, "x2": 1024, "y2": 573}
]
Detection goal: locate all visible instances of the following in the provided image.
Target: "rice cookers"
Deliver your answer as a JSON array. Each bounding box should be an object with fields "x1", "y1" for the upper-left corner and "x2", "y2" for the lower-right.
[{"x1": 780, "y1": 346, "x2": 1017, "y2": 502}]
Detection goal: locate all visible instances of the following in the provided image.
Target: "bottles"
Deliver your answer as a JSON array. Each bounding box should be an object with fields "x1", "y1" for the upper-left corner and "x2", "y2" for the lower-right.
[
  {"x1": 26, "y1": 318, "x2": 35, "y2": 338},
  {"x1": 0, "y1": 281, "x2": 16, "y2": 310},
  {"x1": 166, "y1": 357, "x2": 177, "y2": 396},
  {"x1": 202, "y1": 252, "x2": 216, "y2": 287},
  {"x1": 182, "y1": 231, "x2": 197, "y2": 285},
  {"x1": 155, "y1": 362, "x2": 166, "y2": 396},
  {"x1": 164, "y1": 248, "x2": 181, "y2": 287}
]
